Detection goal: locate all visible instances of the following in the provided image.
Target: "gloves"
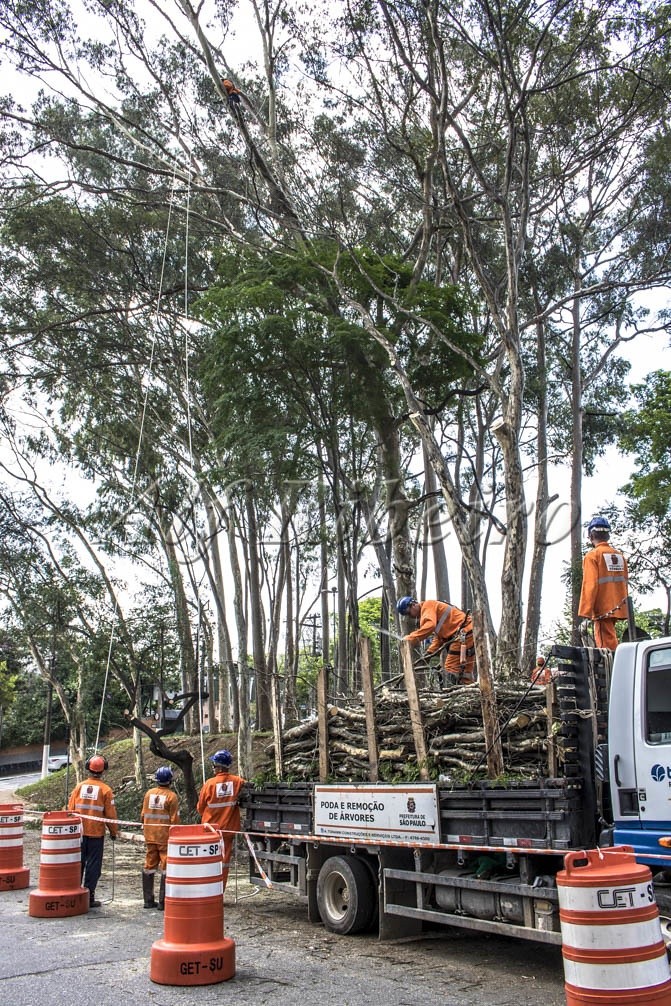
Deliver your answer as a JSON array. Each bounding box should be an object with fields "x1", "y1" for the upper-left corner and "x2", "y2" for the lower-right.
[{"x1": 110, "y1": 835, "x2": 117, "y2": 841}]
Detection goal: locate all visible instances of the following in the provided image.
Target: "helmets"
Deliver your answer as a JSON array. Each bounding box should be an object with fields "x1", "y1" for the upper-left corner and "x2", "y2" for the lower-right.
[
  {"x1": 536, "y1": 657, "x2": 545, "y2": 665},
  {"x1": 85, "y1": 753, "x2": 109, "y2": 773},
  {"x1": 396, "y1": 596, "x2": 414, "y2": 614},
  {"x1": 155, "y1": 767, "x2": 174, "y2": 785},
  {"x1": 208, "y1": 750, "x2": 234, "y2": 768},
  {"x1": 588, "y1": 517, "x2": 611, "y2": 535}
]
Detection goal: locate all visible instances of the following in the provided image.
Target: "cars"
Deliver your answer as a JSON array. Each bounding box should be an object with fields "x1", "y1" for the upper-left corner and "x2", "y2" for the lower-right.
[{"x1": 48, "y1": 747, "x2": 98, "y2": 773}]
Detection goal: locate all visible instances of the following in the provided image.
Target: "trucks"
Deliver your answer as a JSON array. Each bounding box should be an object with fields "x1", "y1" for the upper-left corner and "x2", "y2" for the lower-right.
[{"x1": 236, "y1": 638, "x2": 671, "y2": 958}]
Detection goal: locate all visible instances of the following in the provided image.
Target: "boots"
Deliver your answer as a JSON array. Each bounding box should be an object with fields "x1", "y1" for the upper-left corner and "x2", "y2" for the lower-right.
[
  {"x1": 88, "y1": 890, "x2": 101, "y2": 907},
  {"x1": 143, "y1": 870, "x2": 159, "y2": 909},
  {"x1": 443, "y1": 673, "x2": 459, "y2": 691},
  {"x1": 158, "y1": 873, "x2": 165, "y2": 910}
]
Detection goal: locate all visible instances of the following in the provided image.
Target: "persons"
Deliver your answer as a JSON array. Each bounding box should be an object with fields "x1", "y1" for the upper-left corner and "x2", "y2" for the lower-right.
[
  {"x1": 68, "y1": 754, "x2": 117, "y2": 908},
  {"x1": 578, "y1": 517, "x2": 629, "y2": 651},
  {"x1": 197, "y1": 749, "x2": 248, "y2": 893},
  {"x1": 531, "y1": 657, "x2": 552, "y2": 684},
  {"x1": 140, "y1": 767, "x2": 181, "y2": 911},
  {"x1": 396, "y1": 596, "x2": 476, "y2": 688}
]
